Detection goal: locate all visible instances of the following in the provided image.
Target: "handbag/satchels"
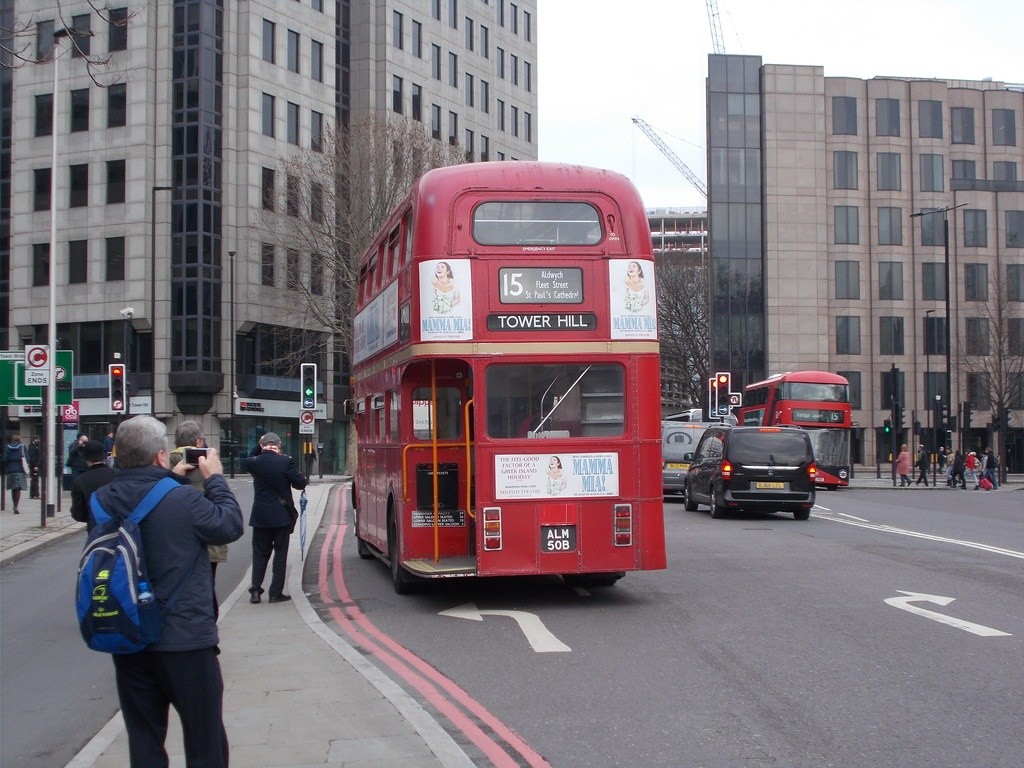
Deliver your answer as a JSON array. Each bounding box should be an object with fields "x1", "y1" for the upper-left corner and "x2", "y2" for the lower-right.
[
  {"x1": 281, "y1": 499, "x2": 300, "y2": 533},
  {"x1": 21, "y1": 445, "x2": 29, "y2": 475}
]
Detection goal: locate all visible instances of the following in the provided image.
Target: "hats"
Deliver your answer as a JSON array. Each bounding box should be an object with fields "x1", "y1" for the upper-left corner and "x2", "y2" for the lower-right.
[
  {"x1": 257, "y1": 432, "x2": 280, "y2": 446},
  {"x1": 78, "y1": 441, "x2": 108, "y2": 461}
]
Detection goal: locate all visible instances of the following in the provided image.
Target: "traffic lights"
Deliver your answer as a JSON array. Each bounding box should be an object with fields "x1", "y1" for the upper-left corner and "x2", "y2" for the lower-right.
[
  {"x1": 108, "y1": 363, "x2": 126, "y2": 412},
  {"x1": 933, "y1": 400, "x2": 948, "y2": 430},
  {"x1": 895, "y1": 407, "x2": 906, "y2": 435},
  {"x1": 300, "y1": 363, "x2": 317, "y2": 411},
  {"x1": 709, "y1": 377, "x2": 722, "y2": 420},
  {"x1": 716, "y1": 371, "x2": 742, "y2": 416},
  {"x1": 963, "y1": 402, "x2": 974, "y2": 433},
  {"x1": 992, "y1": 415, "x2": 1001, "y2": 432},
  {"x1": 1001, "y1": 408, "x2": 1013, "y2": 431},
  {"x1": 883, "y1": 419, "x2": 892, "y2": 438}
]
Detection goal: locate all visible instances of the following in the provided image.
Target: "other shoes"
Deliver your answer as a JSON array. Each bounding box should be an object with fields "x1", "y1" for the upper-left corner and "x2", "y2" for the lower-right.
[
  {"x1": 269, "y1": 594, "x2": 290, "y2": 603},
  {"x1": 250, "y1": 591, "x2": 260, "y2": 602}
]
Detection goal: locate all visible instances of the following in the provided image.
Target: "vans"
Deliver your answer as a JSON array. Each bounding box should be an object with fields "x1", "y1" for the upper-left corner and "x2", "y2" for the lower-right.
[{"x1": 661, "y1": 420, "x2": 722, "y2": 495}]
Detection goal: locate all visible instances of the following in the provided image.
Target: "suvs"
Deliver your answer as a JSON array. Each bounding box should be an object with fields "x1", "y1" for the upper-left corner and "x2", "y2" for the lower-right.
[{"x1": 684, "y1": 423, "x2": 816, "y2": 521}]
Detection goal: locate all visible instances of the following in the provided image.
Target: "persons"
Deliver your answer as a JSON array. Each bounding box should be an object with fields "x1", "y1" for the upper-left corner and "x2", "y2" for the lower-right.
[
  {"x1": 545, "y1": 455, "x2": 575, "y2": 496},
  {"x1": 914, "y1": 444, "x2": 929, "y2": 486},
  {"x1": 167, "y1": 421, "x2": 229, "y2": 621},
  {"x1": 959, "y1": 448, "x2": 980, "y2": 490},
  {"x1": 938, "y1": 447, "x2": 947, "y2": 474},
  {"x1": 951, "y1": 450, "x2": 967, "y2": 489},
  {"x1": 27, "y1": 434, "x2": 41, "y2": 498},
  {"x1": 896, "y1": 443, "x2": 912, "y2": 486},
  {"x1": 3, "y1": 434, "x2": 29, "y2": 514},
  {"x1": 944, "y1": 446, "x2": 957, "y2": 486},
  {"x1": 432, "y1": 261, "x2": 466, "y2": 315},
  {"x1": 70, "y1": 440, "x2": 116, "y2": 524},
  {"x1": 620, "y1": 261, "x2": 654, "y2": 312},
  {"x1": 246, "y1": 432, "x2": 307, "y2": 604},
  {"x1": 88, "y1": 416, "x2": 243, "y2": 768},
  {"x1": 67, "y1": 433, "x2": 88, "y2": 478},
  {"x1": 979, "y1": 447, "x2": 998, "y2": 490},
  {"x1": 304, "y1": 448, "x2": 317, "y2": 477},
  {"x1": 103, "y1": 431, "x2": 114, "y2": 453}
]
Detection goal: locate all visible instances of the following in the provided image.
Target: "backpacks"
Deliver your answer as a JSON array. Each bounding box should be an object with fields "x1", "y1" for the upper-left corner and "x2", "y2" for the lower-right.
[{"x1": 75, "y1": 478, "x2": 182, "y2": 655}]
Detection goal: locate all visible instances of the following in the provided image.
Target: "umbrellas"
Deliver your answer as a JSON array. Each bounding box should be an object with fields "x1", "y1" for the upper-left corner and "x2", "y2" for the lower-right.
[{"x1": 300, "y1": 488, "x2": 308, "y2": 562}]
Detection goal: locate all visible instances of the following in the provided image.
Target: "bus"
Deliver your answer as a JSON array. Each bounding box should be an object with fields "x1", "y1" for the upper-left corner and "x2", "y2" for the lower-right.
[
  {"x1": 738, "y1": 371, "x2": 852, "y2": 490},
  {"x1": 343, "y1": 161, "x2": 667, "y2": 595},
  {"x1": 664, "y1": 408, "x2": 739, "y2": 426}
]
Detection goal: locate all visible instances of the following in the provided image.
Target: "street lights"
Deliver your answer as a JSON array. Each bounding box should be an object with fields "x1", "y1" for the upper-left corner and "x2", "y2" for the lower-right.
[
  {"x1": 909, "y1": 202, "x2": 968, "y2": 453},
  {"x1": 925, "y1": 309, "x2": 936, "y2": 474},
  {"x1": 120, "y1": 307, "x2": 135, "y2": 420},
  {"x1": 45, "y1": 27, "x2": 77, "y2": 519},
  {"x1": 228, "y1": 250, "x2": 237, "y2": 480},
  {"x1": 151, "y1": 186, "x2": 178, "y2": 418}
]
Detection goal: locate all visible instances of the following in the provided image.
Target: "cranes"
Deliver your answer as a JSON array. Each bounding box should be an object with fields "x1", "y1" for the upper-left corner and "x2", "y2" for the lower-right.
[
  {"x1": 705, "y1": 0, "x2": 726, "y2": 54},
  {"x1": 631, "y1": 117, "x2": 707, "y2": 201}
]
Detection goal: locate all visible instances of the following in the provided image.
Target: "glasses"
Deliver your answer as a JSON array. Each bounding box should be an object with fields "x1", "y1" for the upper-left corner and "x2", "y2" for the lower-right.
[{"x1": 195, "y1": 437, "x2": 205, "y2": 444}]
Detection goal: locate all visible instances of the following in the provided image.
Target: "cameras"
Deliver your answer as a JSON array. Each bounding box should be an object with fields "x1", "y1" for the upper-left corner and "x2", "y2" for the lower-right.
[{"x1": 184, "y1": 448, "x2": 209, "y2": 465}]
